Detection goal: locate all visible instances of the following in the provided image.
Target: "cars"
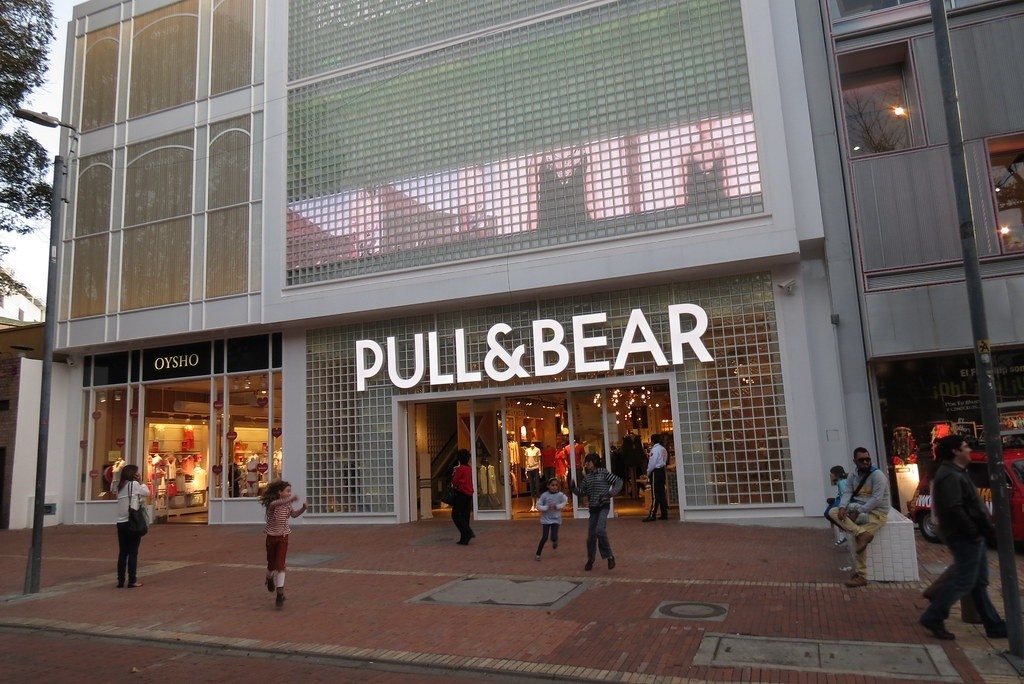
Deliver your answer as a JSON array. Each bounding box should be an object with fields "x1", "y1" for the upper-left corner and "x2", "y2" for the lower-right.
[{"x1": 904, "y1": 447, "x2": 1024, "y2": 543}]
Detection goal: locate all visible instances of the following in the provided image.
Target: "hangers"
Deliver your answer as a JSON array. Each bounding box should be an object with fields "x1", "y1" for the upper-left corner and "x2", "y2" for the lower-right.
[
  {"x1": 164, "y1": 454, "x2": 177, "y2": 461},
  {"x1": 182, "y1": 455, "x2": 194, "y2": 461},
  {"x1": 508, "y1": 440, "x2": 519, "y2": 446}
]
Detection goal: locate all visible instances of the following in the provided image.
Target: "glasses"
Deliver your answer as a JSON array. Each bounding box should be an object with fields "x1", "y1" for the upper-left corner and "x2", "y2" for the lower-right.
[{"x1": 856, "y1": 457, "x2": 872, "y2": 464}]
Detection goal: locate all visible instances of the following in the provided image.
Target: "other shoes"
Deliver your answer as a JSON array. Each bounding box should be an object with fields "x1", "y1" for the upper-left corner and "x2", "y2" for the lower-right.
[
  {"x1": 856, "y1": 532, "x2": 874, "y2": 552},
  {"x1": 608, "y1": 556, "x2": 615, "y2": 569},
  {"x1": 127, "y1": 580, "x2": 143, "y2": 588},
  {"x1": 835, "y1": 538, "x2": 847, "y2": 547},
  {"x1": 117, "y1": 582, "x2": 124, "y2": 587},
  {"x1": 276, "y1": 591, "x2": 285, "y2": 608},
  {"x1": 642, "y1": 516, "x2": 655, "y2": 522},
  {"x1": 843, "y1": 575, "x2": 867, "y2": 588},
  {"x1": 658, "y1": 516, "x2": 667, "y2": 520},
  {"x1": 553, "y1": 540, "x2": 557, "y2": 550},
  {"x1": 457, "y1": 532, "x2": 475, "y2": 544},
  {"x1": 585, "y1": 559, "x2": 595, "y2": 571},
  {"x1": 920, "y1": 613, "x2": 955, "y2": 641},
  {"x1": 536, "y1": 553, "x2": 540, "y2": 560},
  {"x1": 266, "y1": 573, "x2": 275, "y2": 592},
  {"x1": 986, "y1": 622, "x2": 1007, "y2": 638}
]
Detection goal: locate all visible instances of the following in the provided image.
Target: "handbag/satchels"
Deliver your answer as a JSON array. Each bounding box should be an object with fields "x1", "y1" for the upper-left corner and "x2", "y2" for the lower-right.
[
  {"x1": 128, "y1": 506, "x2": 147, "y2": 536},
  {"x1": 441, "y1": 486, "x2": 455, "y2": 506}
]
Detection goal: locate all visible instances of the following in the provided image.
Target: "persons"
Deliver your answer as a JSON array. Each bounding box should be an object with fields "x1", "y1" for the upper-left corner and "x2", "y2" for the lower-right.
[
  {"x1": 150, "y1": 453, "x2": 162, "y2": 484},
  {"x1": 570, "y1": 453, "x2": 623, "y2": 571},
  {"x1": 110, "y1": 457, "x2": 125, "y2": 494},
  {"x1": 257, "y1": 481, "x2": 309, "y2": 607},
  {"x1": 116, "y1": 465, "x2": 149, "y2": 588},
  {"x1": 184, "y1": 424, "x2": 194, "y2": 450},
  {"x1": 642, "y1": 433, "x2": 668, "y2": 522},
  {"x1": 918, "y1": 435, "x2": 1008, "y2": 641},
  {"x1": 824, "y1": 465, "x2": 849, "y2": 545},
  {"x1": 536, "y1": 476, "x2": 568, "y2": 561},
  {"x1": 829, "y1": 446, "x2": 890, "y2": 587},
  {"x1": 245, "y1": 457, "x2": 257, "y2": 496},
  {"x1": 153, "y1": 423, "x2": 165, "y2": 449},
  {"x1": 227, "y1": 463, "x2": 240, "y2": 497},
  {"x1": 524, "y1": 440, "x2": 586, "y2": 512},
  {"x1": 441, "y1": 448, "x2": 476, "y2": 545}
]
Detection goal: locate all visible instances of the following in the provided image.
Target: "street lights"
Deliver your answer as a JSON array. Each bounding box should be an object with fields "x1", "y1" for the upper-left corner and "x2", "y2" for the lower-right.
[{"x1": 13, "y1": 107, "x2": 79, "y2": 594}]
